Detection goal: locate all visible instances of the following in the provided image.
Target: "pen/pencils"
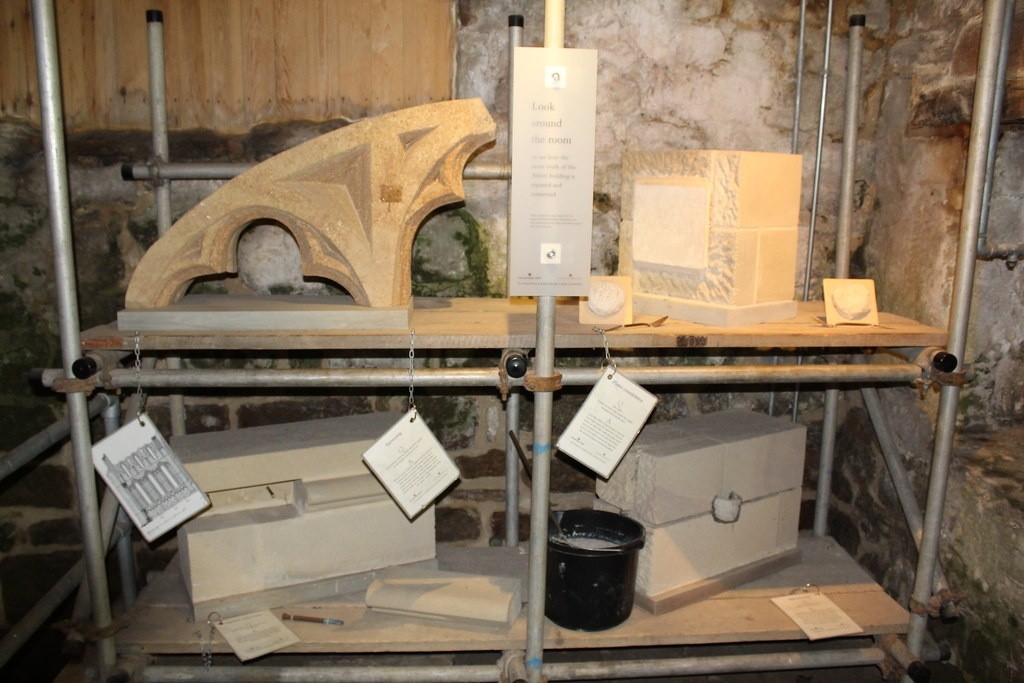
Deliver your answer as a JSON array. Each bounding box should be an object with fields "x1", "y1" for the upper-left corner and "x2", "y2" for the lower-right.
[{"x1": 282, "y1": 612, "x2": 344, "y2": 625}]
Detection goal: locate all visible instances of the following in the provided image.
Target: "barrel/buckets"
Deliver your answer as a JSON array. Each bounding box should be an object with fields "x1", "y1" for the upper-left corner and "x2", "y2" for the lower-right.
[{"x1": 544, "y1": 509, "x2": 646, "y2": 632}]
